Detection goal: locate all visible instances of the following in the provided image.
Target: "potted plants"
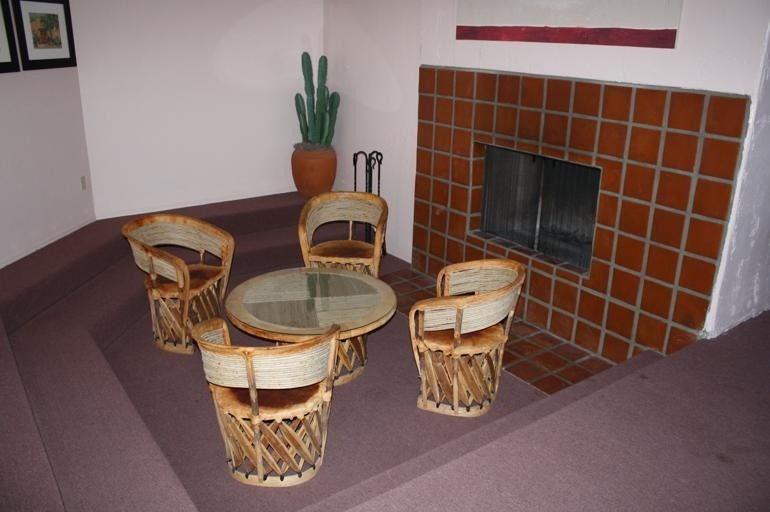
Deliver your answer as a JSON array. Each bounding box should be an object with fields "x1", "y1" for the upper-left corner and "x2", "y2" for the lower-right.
[{"x1": 291, "y1": 52, "x2": 340, "y2": 198}]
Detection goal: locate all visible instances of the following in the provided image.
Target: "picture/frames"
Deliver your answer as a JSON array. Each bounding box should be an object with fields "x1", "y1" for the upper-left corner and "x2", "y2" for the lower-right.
[
  {"x1": 12, "y1": 0, "x2": 77, "y2": 71},
  {"x1": 0, "y1": 0, "x2": 20, "y2": 73}
]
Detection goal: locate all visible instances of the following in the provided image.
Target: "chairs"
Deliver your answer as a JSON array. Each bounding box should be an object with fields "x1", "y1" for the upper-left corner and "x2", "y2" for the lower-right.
[
  {"x1": 190, "y1": 318, "x2": 340, "y2": 488},
  {"x1": 121, "y1": 213, "x2": 236, "y2": 355},
  {"x1": 298, "y1": 192, "x2": 388, "y2": 277},
  {"x1": 408, "y1": 259, "x2": 526, "y2": 418}
]
